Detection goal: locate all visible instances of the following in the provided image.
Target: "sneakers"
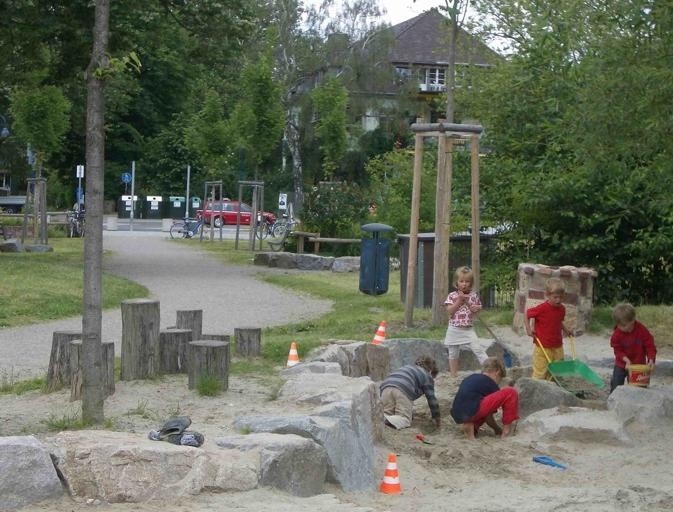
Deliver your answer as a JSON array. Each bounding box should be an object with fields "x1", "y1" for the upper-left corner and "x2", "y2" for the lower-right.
[{"x1": 149, "y1": 416, "x2": 204, "y2": 447}]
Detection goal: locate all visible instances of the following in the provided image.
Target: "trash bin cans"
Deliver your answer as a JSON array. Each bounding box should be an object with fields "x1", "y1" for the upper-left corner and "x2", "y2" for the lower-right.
[{"x1": 360, "y1": 224, "x2": 393, "y2": 296}]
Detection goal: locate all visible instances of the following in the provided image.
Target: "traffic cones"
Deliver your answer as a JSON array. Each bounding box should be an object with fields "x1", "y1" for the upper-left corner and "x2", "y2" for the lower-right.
[
  {"x1": 379, "y1": 452, "x2": 402, "y2": 495},
  {"x1": 371, "y1": 320, "x2": 387, "y2": 344},
  {"x1": 286, "y1": 340, "x2": 299, "y2": 367}
]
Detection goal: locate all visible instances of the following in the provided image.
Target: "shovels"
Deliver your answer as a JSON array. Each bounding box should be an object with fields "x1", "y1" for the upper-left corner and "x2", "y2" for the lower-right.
[{"x1": 457, "y1": 290, "x2": 512, "y2": 369}]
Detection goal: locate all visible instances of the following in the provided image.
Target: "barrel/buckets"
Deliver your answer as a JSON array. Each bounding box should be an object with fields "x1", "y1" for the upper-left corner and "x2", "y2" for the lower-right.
[{"x1": 627, "y1": 364, "x2": 651, "y2": 387}]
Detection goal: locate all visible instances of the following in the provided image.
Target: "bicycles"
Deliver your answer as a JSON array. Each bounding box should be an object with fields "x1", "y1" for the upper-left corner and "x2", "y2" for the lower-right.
[
  {"x1": 256, "y1": 214, "x2": 301, "y2": 241},
  {"x1": 170, "y1": 217, "x2": 211, "y2": 240},
  {"x1": 67, "y1": 210, "x2": 85, "y2": 238}
]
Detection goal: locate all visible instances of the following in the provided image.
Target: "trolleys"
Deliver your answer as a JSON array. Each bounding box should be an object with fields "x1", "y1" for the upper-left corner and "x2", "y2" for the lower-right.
[{"x1": 535, "y1": 336, "x2": 605, "y2": 398}]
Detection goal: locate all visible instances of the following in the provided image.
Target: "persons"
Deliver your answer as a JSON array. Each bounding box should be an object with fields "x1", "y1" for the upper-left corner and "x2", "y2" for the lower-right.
[
  {"x1": 609, "y1": 303, "x2": 657, "y2": 396},
  {"x1": 379, "y1": 354, "x2": 441, "y2": 431},
  {"x1": 523, "y1": 277, "x2": 574, "y2": 383},
  {"x1": 279, "y1": 197, "x2": 285, "y2": 205},
  {"x1": 443, "y1": 265, "x2": 489, "y2": 377},
  {"x1": 451, "y1": 356, "x2": 520, "y2": 440}
]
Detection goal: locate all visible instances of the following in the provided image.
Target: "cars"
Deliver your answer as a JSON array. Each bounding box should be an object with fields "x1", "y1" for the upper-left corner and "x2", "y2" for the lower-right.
[{"x1": 197, "y1": 200, "x2": 276, "y2": 228}]
